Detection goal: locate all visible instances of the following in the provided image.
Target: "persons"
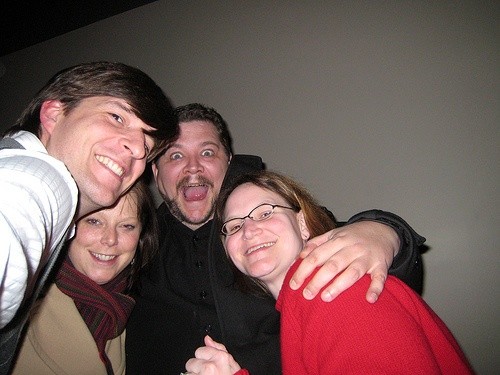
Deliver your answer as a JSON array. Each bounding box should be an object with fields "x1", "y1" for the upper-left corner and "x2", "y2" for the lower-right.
[
  {"x1": 179, "y1": 166, "x2": 475, "y2": 375},
  {"x1": 124, "y1": 101, "x2": 428, "y2": 375},
  {"x1": 0, "y1": 59, "x2": 181, "y2": 375},
  {"x1": 5, "y1": 174, "x2": 156, "y2": 375}
]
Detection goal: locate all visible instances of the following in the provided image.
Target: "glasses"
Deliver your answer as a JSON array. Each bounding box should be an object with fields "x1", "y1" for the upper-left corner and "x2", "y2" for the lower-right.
[{"x1": 218, "y1": 203, "x2": 297, "y2": 237}]
